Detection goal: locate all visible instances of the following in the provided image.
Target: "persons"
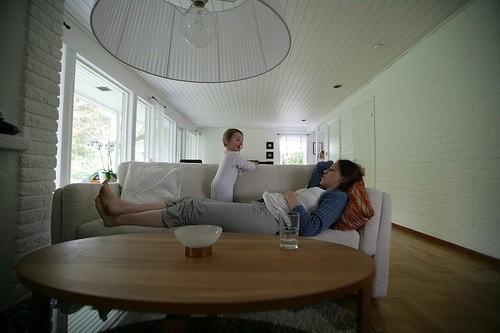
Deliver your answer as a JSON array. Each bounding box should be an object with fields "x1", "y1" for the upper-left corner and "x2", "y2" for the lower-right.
[
  {"x1": 95, "y1": 159, "x2": 366, "y2": 236},
  {"x1": 210, "y1": 129, "x2": 257, "y2": 202}
]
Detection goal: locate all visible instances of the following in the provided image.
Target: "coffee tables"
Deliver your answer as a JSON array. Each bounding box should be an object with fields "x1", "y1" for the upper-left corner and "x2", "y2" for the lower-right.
[{"x1": 15, "y1": 232, "x2": 377, "y2": 333}]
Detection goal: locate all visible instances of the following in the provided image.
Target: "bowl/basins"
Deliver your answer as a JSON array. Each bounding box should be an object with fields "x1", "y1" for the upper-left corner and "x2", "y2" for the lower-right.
[{"x1": 174, "y1": 225, "x2": 222, "y2": 248}]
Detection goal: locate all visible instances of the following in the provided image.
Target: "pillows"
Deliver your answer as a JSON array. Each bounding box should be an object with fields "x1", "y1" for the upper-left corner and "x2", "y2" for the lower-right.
[
  {"x1": 328, "y1": 178, "x2": 377, "y2": 231},
  {"x1": 120, "y1": 163, "x2": 186, "y2": 202}
]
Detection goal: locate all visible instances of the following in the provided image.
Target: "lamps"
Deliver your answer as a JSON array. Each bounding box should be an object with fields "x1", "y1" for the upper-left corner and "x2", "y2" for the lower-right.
[{"x1": 90, "y1": 0, "x2": 292, "y2": 83}]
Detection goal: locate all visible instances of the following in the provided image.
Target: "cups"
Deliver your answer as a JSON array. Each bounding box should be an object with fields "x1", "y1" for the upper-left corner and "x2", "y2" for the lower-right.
[{"x1": 279, "y1": 212, "x2": 300, "y2": 249}]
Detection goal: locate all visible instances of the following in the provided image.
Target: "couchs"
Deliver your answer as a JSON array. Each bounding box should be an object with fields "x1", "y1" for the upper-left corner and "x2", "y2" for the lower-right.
[{"x1": 51, "y1": 160, "x2": 393, "y2": 306}]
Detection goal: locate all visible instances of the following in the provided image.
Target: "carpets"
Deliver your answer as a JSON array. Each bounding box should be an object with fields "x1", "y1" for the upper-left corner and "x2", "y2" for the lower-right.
[{"x1": 112, "y1": 310, "x2": 384, "y2": 333}]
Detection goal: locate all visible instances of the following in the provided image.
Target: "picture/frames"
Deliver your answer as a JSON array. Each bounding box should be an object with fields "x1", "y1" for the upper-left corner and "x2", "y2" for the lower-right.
[
  {"x1": 265, "y1": 152, "x2": 274, "y2": 159},
  {"x1": 266, "y1": 141, "x2": 274, "y2": 149}
]
